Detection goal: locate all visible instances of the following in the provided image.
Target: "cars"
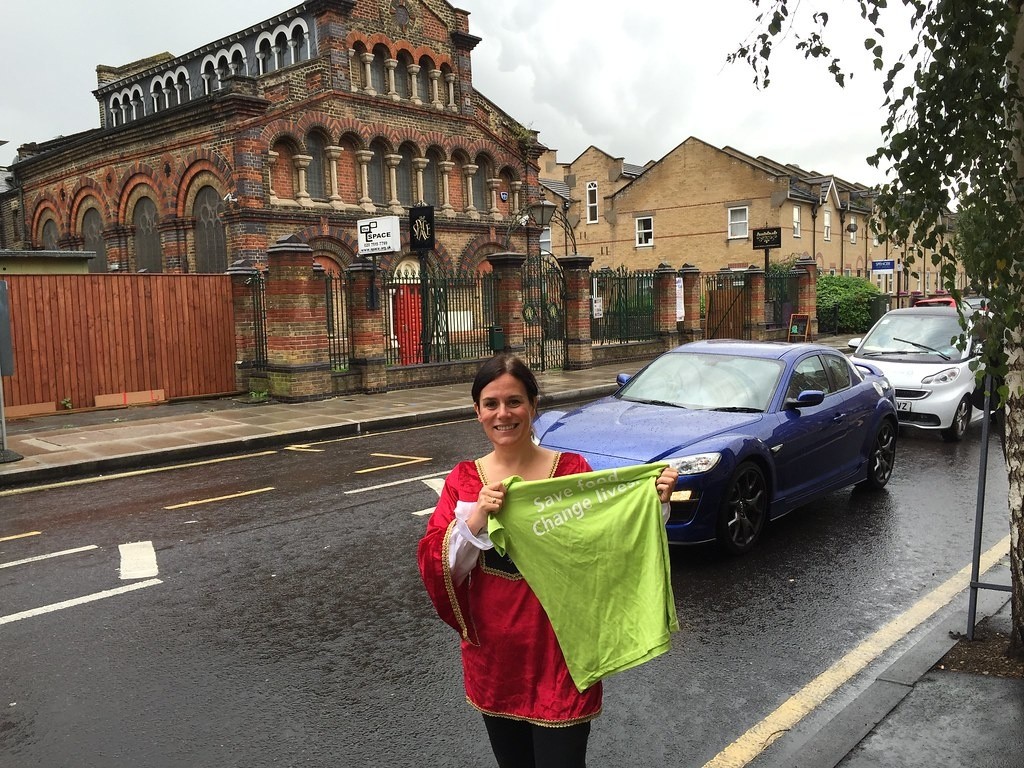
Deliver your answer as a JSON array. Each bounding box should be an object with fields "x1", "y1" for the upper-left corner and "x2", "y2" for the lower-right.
[
  {"x1": 912, "y1": 296, "x2": 957, "y2": 307},
  {"x1": 962, "y1": 296, "x2": 991, "y2": 311},
  {"x1": 847, "y1": 305, "x2": 994, "y2": 443}
]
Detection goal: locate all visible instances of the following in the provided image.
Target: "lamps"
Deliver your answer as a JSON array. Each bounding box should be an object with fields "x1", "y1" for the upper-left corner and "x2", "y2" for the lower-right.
[{"x1": 530, "y1": 188, "x2": 557, "y2": 226}]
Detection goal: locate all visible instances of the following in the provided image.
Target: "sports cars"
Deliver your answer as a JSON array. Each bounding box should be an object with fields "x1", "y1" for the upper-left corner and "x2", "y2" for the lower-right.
[{"x1": 530, "y1": 338, "x2": 898, "y2": 561}]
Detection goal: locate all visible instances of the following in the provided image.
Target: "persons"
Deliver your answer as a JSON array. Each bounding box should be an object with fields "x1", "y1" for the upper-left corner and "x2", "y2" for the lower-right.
[{"x1": 416, "y1": 353, "x2": 678, "y2": 768}]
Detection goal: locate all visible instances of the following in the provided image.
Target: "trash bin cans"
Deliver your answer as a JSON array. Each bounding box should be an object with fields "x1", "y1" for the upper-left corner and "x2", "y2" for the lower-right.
[{"x1": 867, "y1": 291, "x2": 892, "y2": 334}]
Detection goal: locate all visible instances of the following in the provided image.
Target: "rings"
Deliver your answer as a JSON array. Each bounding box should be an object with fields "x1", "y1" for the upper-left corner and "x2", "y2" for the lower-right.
[{"x1": 492, "y1": 498, "x2": 497, "y2": 503}]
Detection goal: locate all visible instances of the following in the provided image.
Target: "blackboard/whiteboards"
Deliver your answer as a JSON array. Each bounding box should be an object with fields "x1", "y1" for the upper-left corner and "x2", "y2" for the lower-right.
[{"x1": 788, "y1": 314, "x2": 811, "y2": 336}]
